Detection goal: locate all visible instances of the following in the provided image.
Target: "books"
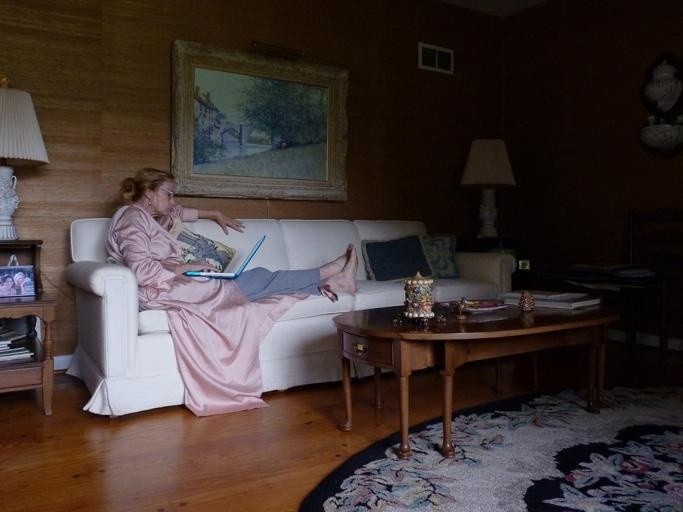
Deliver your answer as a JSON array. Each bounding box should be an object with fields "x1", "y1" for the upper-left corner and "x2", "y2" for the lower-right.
[
  {"x1": 498, "y1": 291, "x2": 601, "y2": 309},
  {"x1": 0, "y1": 325, "x2": 35, "y2": 362}
]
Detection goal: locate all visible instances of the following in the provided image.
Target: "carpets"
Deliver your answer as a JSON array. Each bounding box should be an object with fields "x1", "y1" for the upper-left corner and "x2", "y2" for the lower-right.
[{"x1": 298, "y1": 380, "x2": 682, "y2": 512}]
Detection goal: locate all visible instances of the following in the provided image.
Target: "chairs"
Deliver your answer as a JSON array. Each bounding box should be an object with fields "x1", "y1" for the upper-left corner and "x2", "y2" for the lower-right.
[{"x1": 619, "y1": 203, "x2": 683, "y2": 387}]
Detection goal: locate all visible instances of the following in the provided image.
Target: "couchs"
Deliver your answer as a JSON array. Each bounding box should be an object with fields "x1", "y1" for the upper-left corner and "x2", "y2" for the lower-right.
[{"x1": 65, "y1": 217, "x2": 519, "y2": 418}]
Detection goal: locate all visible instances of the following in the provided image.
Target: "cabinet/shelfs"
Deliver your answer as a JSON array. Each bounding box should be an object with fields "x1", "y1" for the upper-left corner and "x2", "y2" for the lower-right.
[{"x1": 1, "y1": 237, "x2": 58, "y2": 418}]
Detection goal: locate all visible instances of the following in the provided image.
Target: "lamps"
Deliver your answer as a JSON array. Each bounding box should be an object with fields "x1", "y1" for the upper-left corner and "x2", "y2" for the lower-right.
[
  {"x1": 0, "y1": 88, "x2": 51, "y2": 240},
  {"x1": 460, "y1": 136, "x2": 518, "y2": 237}
]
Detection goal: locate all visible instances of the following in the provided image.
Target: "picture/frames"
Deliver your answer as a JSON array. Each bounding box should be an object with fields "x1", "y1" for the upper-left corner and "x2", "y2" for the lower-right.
[
  {"x1": 0, "y1": 264, "x2": 38, "y2": 300},
  {"x1": 169, "y1": 39, "x2": 352, "y2": 203}
]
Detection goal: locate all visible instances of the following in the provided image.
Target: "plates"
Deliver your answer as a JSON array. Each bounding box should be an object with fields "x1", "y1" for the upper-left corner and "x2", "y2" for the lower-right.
[{"x1": 465, "y1": 304, "x2": 509, "y2": 314}]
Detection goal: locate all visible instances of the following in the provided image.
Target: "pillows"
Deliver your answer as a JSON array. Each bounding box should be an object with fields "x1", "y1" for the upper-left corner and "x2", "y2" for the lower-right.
[
  {"x1": 361, "y1": 233, "x2": 434, "y2": 282},
  {"x1": 419, "y1": 230, "x2": 460, "y2": 277}
]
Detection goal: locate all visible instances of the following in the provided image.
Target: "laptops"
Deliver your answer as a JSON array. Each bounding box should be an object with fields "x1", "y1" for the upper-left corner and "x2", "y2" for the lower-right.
[{"x1": 183, "y1": 235, "x2": 266, "y2": 279}]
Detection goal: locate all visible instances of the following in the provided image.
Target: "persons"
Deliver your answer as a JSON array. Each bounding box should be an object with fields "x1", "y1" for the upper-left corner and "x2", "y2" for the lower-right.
[
  {"x1": 0, "y1": 271, "x2": 31, "y2": 297},
  {"x1": 106, "y1": 167, "x2": 359, "y2": 311}
]
